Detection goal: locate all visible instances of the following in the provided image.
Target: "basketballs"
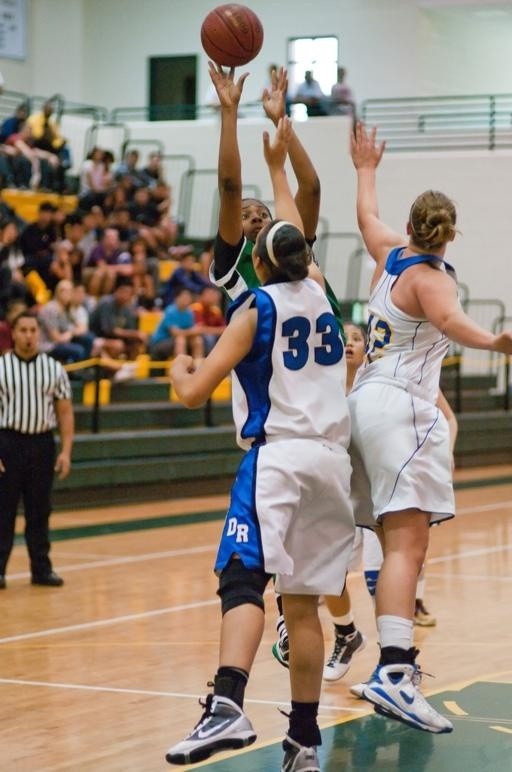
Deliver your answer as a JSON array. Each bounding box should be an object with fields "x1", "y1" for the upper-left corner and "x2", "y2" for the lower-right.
[{"x1": 202, "y1": 3, "x2": 264, "y2": 68}]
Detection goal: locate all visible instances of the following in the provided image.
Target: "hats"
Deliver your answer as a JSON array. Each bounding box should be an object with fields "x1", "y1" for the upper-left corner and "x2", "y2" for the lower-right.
[
  {"x1": 38, "y1": 199, "x2": 58, "y2": 213},
  {"x1": 63, "y1": 214, "x2": 85, "y2": 226}
]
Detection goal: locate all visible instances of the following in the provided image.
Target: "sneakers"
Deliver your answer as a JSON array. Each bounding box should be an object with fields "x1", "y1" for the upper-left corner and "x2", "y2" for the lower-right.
[
  {"x1": 324, "y1": 630, "x2": 364, "y2": 679},
  {"x1": 31, "y1": 569, "x2": 64, "y2": 588},
  {"x1": 278, "y1": 733, "x2": 324, "y2": 772},
  {"x1": 363, "y1": 661, "x2": 453, "y2": 733},
  {"x1": 167, "y1": 694, "x2": 257, "y2": 764},
  {"x1": 414, "y1": 596, "x2": 436, "y2": 628}
]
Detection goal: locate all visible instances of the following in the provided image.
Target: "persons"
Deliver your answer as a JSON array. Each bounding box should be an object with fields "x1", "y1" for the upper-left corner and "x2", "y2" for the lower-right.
[
  {"x1": 2, "y1": 272, "x2": 145, "y2": 382},
  {"x1": 1, "y1": 100, "x2": 71, "y2": 190},
  {"x1": 325, "y1": 321, "x2": 384, "y2": 698},
  {"x1": 166, "y1": 115, "x2": 355, "y2": 772},
  {"x1": 79, "y1": 148, "x2": 188, "y2": 259},
  {"x1": 1, "y1": 196, "x2": 167, "y2": 311},
  {"x1": 145, "y1": 246, "x2": 227, "y2": 361},
  {"x1": 1, "y1": 313, "x2": 74, "y2": 587},
  {"x1": 260, "y1": 60, "x2": 358, "y2": 116},
  {"x1": 352, "y1": 122, "x2": 512, "y2": 734},
  {"x1": 413, "y1": 392, "x2": 458, "y2": 626},
  {"x1": 205, "y1": 57, "x2": 352, "y2": 668}
]
